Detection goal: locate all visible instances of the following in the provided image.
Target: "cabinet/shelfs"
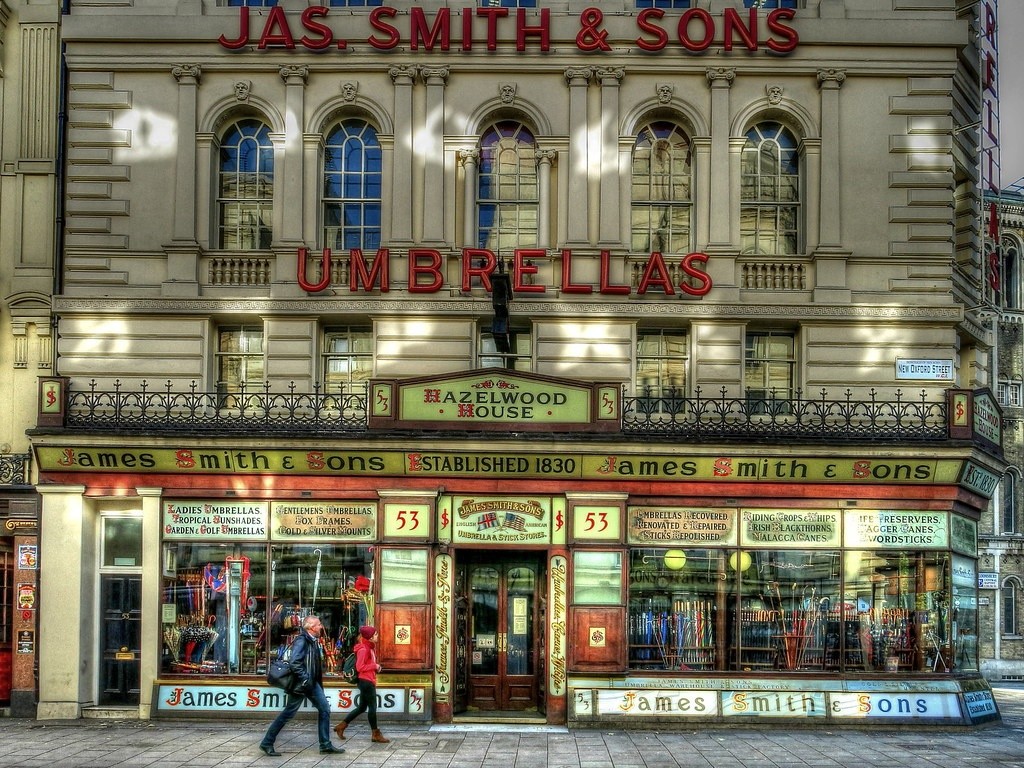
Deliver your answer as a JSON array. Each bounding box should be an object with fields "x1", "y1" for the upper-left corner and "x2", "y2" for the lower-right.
[
  {"x1": 240, "y1": 641, "x2": 261, "y2": 675},
  {"x1": 628, "y1": 616, "x2": 953, "y2": 672}
]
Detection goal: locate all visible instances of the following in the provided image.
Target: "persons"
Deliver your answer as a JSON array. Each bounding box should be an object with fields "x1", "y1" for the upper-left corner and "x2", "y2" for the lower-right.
[
  {"x1": 333, "y1": 626, "x2": 389, "y2": 743},
  {"x1": 259, "y1": 615, "x2": 344, "y2": 756}
]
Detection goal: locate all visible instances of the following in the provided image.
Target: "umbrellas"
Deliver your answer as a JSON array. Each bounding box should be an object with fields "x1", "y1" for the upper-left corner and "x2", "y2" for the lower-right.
[
  {"x1": 628, "y1": 599, "x2": 715, "y2": 671},
  {"x1": 164, "y1": 556, "x2": 356, "y2": 675},
  {"x1": 738, "y1": 581, "x2": 918, "y2": 671}
]
